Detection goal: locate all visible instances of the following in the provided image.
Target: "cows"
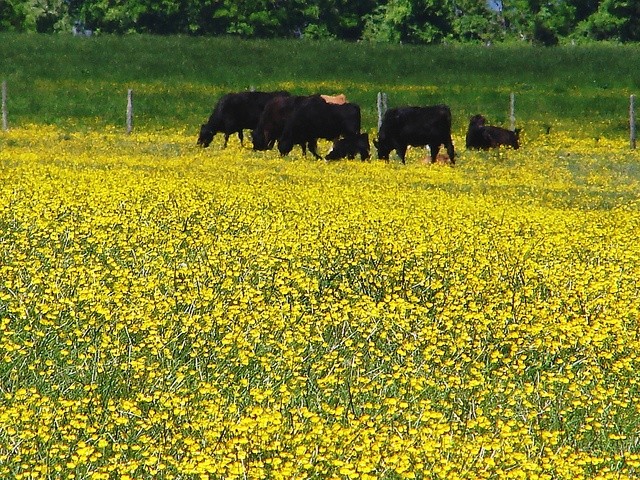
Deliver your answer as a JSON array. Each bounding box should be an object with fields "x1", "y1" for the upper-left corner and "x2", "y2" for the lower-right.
[
  {"x1": 197, "y1": 89, "x2": 290, "y2": 149},
  {"x1": 278, "y1": 94, "x2": 361, "y2": 156},
  {"x1": 324, "y1": 133, "x2": 370, "y2": 164},
  {"x1": 465, "y1": 126, "x2": 521, "y2": 150},
  {"x1": 250, "y1": 95, "x2": 311, "y2": 150},
  {"x1": 373, "y1": 104, "x2": 455, "y2": 165},
  {"x1": 465, "y1": 114, "x2": 486, "y2": 151}
]
